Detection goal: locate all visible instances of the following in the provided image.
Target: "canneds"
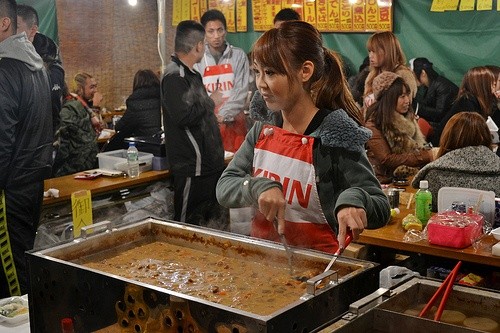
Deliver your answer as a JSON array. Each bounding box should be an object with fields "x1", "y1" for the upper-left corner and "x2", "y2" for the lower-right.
[{"x1": 386, "y1": 188, "x2": 399, "y2": 209}]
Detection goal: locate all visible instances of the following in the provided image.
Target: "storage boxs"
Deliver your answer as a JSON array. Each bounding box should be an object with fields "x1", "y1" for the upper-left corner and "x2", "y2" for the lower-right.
[
  {"x1": 96, "y1": 149, "x2": 154, "y2": 174},
  {"x1": 428, "y1": 212, "x2": 483, "y2": 249}
]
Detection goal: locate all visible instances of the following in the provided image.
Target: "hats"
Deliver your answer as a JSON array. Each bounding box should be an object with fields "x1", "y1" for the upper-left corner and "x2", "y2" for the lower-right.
[{"x1": 372, "y1": 71, "x2": 401, "y2": 102}]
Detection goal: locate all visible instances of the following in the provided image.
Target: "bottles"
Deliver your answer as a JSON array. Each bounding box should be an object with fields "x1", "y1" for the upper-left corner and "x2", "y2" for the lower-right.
[
  {"x1": 402, "y1": 214, "x2": 423, "y2": 231},
  {"x1": 127, "y1": 141, "x2": 139, "y2": 178},
  {"x1": 414, "y1": 180, "x2": 432, "y2": 227}
]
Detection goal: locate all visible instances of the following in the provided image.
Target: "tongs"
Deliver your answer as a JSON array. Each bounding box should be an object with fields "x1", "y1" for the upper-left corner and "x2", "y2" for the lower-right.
[{"x1": 418, "y1": 261, "x2": 462, "y2": 322}]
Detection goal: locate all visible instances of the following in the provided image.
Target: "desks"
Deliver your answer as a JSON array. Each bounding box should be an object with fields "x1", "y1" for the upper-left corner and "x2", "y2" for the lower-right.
[
  {"x1": 40, "y1": 169, "x2": 169, "y2": 241},
  {"x1": 351, "y1": 205, "x2": 500, "y2": 267}
]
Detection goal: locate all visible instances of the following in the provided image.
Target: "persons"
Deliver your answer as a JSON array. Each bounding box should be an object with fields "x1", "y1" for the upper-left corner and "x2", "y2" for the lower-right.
[
  {"x1": 273, "y1": 8, "x2": 301, "y2": 29},
  {"x1": 216, "y1": 20, "x2": 391, "y2": 255},
  {"x1": 161, "y1": 21, "x2": 224, "y2": 226},
  {"x1": 412, "y1": 111, "x2": 500, "y2": 202},
  {"x1": 0, "y1": 0, "x2": 53, "y2": 299},
  {"x1": 16, "y1": 5, "x2": 65, "y2": 98},
  {"x1": 348, "y1": 31, "x2": 500, "y2": 148},
  {"x1": 363, "y1": 71, "x2": 433, "y2": 184},
  {"x1": 193, "y1": 10, "x2": 250, "y2": 154},
  {"x1": 67, "y1": 73, "x2": 102, "y2": 126},
  {"x1": 114, "y1": 69, "x2": 161, "y2": 157}
]
